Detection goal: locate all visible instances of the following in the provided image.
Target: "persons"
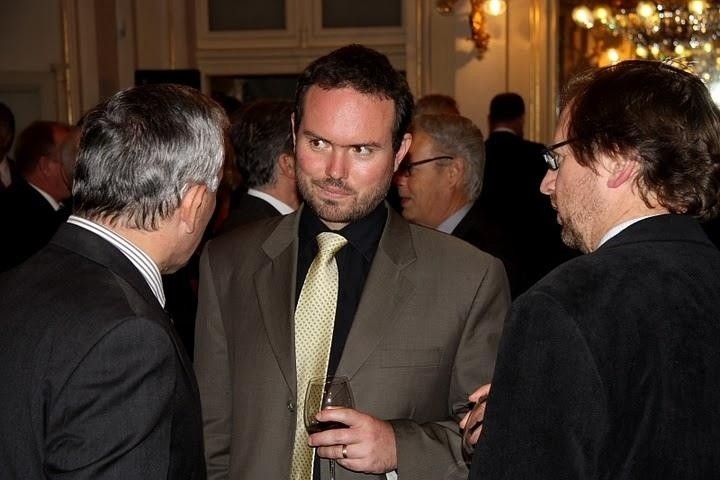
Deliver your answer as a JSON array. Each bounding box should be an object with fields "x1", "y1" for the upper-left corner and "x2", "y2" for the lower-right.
[
  {"x1": 0, "y1": 83, "x2": 231, "y2": 480},
  {"x1": 455, "y1": 60, "x2": 720, "y2": 480},
  {"x1": 1, "y1": 92, "x2": 578, "y2": 344},
  {"x1": 191, "y1": 42, "x2": 513, "y2": 480}
]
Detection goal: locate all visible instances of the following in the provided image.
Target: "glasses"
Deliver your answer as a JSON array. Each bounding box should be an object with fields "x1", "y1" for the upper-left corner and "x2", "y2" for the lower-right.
[
  {"x1": 396, "y1": 156, "x2": 454, "y2": 177},
  {"x1": 540, "y1": 135, "x2": 581, "y2": 171}
]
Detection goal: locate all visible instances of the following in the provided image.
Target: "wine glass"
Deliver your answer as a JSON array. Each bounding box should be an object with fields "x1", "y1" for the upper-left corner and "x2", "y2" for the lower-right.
[{"x1": 304, "y1": 375, "x2": 355, "y2": 480}]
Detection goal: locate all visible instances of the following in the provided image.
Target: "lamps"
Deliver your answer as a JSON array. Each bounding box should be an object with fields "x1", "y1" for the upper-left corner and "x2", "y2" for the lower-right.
[
  {"x1": 570, "y1": 0, "x2": 720, "y2": 83},
  {"x1": 433, "y1": 0, "x2": 508, "y2": 63}
]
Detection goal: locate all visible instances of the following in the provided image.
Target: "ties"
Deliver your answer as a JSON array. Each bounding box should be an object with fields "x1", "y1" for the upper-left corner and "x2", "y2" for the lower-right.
[{"x1": 290, "y1": 231, "x2": 348, "y2": 479}]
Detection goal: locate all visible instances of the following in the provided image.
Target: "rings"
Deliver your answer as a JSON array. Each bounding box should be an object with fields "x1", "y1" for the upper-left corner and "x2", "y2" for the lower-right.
[{"x1": 342, "y1": 444, "x2": 349, "y2": 459}]
